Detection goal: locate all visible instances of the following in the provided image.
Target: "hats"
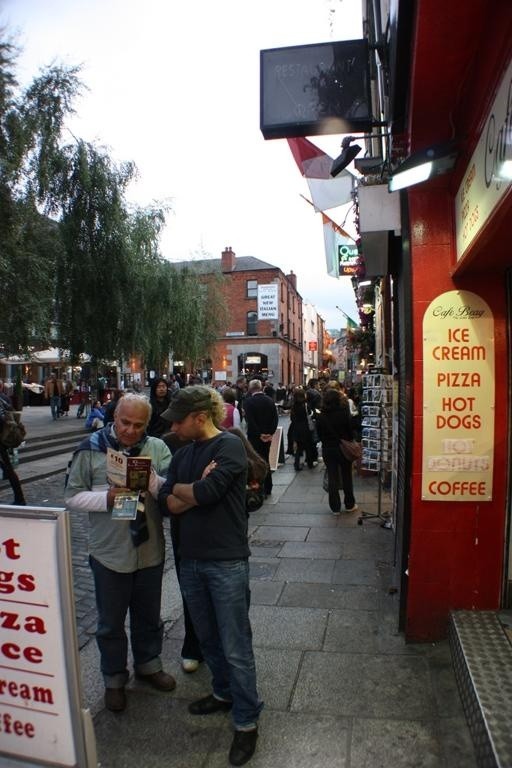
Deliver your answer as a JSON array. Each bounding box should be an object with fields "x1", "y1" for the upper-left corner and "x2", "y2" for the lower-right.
[{"x1": 160, "y1": 387, "x2": 213, "y2": 422}]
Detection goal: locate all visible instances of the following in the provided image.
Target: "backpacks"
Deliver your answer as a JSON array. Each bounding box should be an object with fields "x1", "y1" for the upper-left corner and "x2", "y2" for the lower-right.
[{"x1": 0, "y1": 409, "x2": 27, "y2": 449}]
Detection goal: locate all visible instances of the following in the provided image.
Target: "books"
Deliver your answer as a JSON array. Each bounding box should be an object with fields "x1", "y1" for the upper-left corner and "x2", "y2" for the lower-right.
[{"x1": 107, "y1": 448, "x2": 152, "y2": 522}]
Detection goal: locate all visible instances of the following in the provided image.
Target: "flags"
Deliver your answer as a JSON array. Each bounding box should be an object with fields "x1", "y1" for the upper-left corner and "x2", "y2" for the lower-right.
[
  {"x1": 347, "y1": 317, "x2": 357, "y2": 338},
  {"x1": 287, "y1": 137, "x2": 357, "y2": 215},
  {"x1": 323, "y1": 213, "x2": 351, "y2": 280}
]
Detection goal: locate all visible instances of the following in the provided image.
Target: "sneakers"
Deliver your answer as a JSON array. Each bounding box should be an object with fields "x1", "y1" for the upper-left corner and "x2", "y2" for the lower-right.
[
  {"x1": 133, "y1": 670, "x2": 176, "y2": 691},
  {"x1": 329, "y1": 509, "x2": 341, "y2": 516},
  {"x1": 183, "y1": 659, "x2": 199, "y2": 673},
  {"x1": 343, "y1": 504, "x2": 358, "y2": 512},
  {"x1": 104, "y1": 688, "x2": 130, "y2": 713}
]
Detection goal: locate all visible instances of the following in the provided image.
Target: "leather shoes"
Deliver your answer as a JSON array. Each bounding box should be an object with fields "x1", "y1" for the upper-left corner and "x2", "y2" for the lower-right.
[
  {"x1": 187, "y1": 695, "x2": 233, "y2": 716},
  {"x1": 228, "y1": 724, "x2": 259, "y2": 764}
]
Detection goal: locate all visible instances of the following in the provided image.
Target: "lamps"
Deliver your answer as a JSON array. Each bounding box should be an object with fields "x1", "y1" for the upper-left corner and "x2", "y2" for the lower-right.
[
  {"x1": 387, "y1": 139, "x2": 462, "y2": 194},
  {"x1": 330, "y1": 134, "x2": 390, "y2": 177}
]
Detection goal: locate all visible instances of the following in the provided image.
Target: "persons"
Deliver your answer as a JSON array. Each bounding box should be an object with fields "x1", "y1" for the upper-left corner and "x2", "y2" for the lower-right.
[
  {"x1": 45, "y1": 372, "x2": 74, "y2": 421},
  {"x1": 189, "y1": 368, "x2": 204, "y2": 384},
  {"x1": 243, "y1": 379, "x2": 278, "y2": 500},
  {"x1": 77, "y1": 371, "x2": 107, "y2": 420},
  {"x1": 161, "y1": 383, "x2": 268, "y2": 671},
  {"x1": 216, "y1": 374, "x2": 329, "y2": 470},
  {"x1": 65, "y1": 391, "x2": 175, "y2": 708},
  {"x1": 2, "y1": 381, "x2": 27, "y2": 505},
  {"x1": 134, "y1": 373, "x2": 184, "y2": 438},
  {"x1": 315, "y1": 380, "x2": 363, "y2": 516},
  {"x1": 158, "y1": 389, "x2": 263, "y2": 766}
]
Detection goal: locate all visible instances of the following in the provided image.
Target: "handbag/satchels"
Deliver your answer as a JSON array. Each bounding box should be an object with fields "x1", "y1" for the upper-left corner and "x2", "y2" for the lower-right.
[
  {"x1": 338, "y1": 438, "x2": 361, "y2": 462},
  {"x1": 306, "y1": 409, "x2": 316, "y2": 431}
]
